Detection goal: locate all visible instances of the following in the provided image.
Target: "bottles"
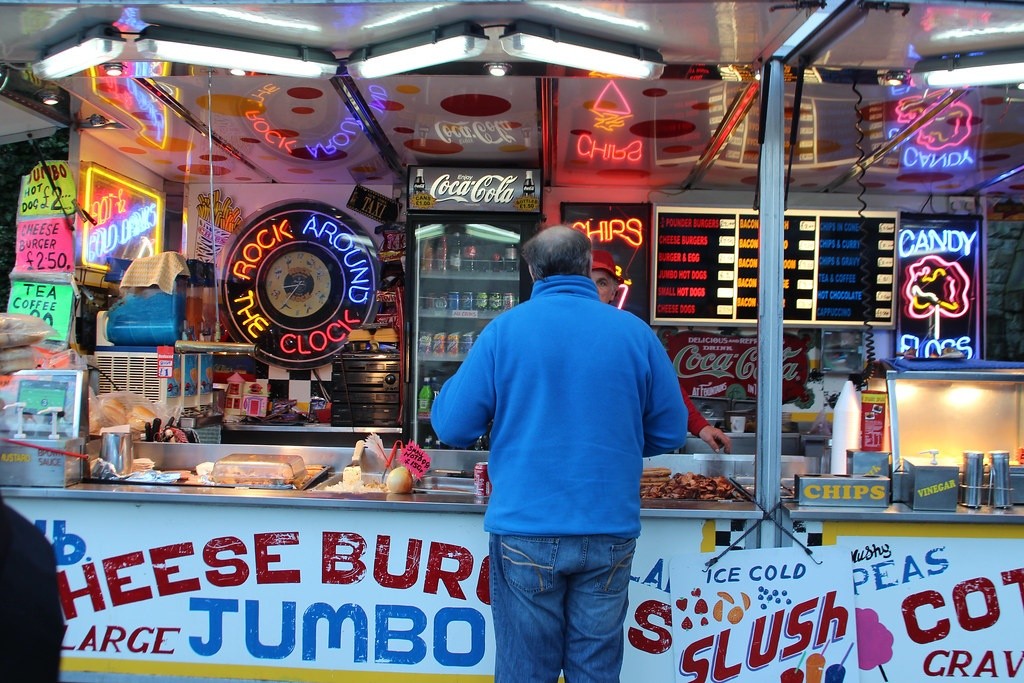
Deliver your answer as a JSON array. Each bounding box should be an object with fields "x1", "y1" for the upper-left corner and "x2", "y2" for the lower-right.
[
  {"x1": 463, "y1": 240, "x2": 476, "y2": 271},
  {"x1": 490, "y1": 254, "x2": 503, "y2": 273},
  {"x1": 431, "y1": 377, "x2": 440, "y2": 402},
  {"x1": 421, "y1": 238, "x2": 432, "y2": 273},
  {"x1": 961, "y1": 451, "x2": 984, "y2": 507},
  {"x1": 505, "y1": 244, "x2": 517, "y2": 272},
  {"x1": 475, "y1": 435, "x2": 488, "y2": 450},
  {"x1": 523, "y1": 171, "x2": 535, "y2": 197},
  {"x1": 436, "y1": 236, "x2": 447, "y2": 273},
  {"x1": 449, "y1": 232, "x2": 461, "y2": 273},
  {"x1": 413, "y1": 169, "x2": 424, "y2": 194},
  {"x1": 423, "y1": 435, "x2": 441, "y2": 449},
  {"x1": 418, "y1": 377, "x2": 431, "y2": 412},
  {"x1": 988, "y1": 451, "x2": 1011, "y2": 507}
]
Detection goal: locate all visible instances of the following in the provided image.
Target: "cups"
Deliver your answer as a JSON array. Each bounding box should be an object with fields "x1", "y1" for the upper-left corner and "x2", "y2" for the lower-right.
[
  {"x1": 730, "y1": 416, "x2": 746, "y2": 433},
  {"x1": 521, "y1": 127, "x2": 532, "y2": 147},
  {"x1": 418, "y1": 127, "x2": 429, "y2": 147}
]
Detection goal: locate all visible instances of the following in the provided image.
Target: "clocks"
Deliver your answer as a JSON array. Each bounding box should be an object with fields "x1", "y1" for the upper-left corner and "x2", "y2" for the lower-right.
[{"x1": 255, "y1": 240, "x2": 345, "y2": 332}]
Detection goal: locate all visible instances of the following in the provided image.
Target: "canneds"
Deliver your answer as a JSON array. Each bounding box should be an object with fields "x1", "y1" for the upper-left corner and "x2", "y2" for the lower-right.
[
  {"x1": 474, "y1": 462, "x2": 493, "y2": 498},
  {"x1": 426, "y1": 291, "x2": 517, "y2": 310},
  {"x1": 419, "y1": 329, "x2": 478, "y2": 354}
]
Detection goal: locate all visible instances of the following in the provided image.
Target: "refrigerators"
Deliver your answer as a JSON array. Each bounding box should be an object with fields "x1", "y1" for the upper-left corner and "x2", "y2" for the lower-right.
[{"x1": 402, "y1": 164, "x2": 544, "y2": 451}]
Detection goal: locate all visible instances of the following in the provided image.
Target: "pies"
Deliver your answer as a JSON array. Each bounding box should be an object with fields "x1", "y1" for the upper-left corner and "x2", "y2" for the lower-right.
[{"x1": 640, "y1": 468, "x2": 672, "y2": 491}]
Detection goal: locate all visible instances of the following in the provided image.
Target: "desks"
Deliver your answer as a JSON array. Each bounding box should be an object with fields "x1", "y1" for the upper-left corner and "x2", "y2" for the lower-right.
[{"x1": 222, "y1": 425, "x2": 404, "y2": 446}]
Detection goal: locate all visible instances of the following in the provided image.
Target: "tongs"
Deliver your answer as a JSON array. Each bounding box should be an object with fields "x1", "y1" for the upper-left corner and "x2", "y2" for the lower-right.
[
  {"x1": 381, "y1": 440, "x2": 404, "y2": 483},
  {"x1": 727, "y1": 475, "x2": 755, "y2": 503}
]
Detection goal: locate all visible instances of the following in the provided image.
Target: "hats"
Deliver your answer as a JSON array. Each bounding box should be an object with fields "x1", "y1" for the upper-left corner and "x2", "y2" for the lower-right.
[{"x1": 591, "y1": 250, "x2": 616, "y2": 276}]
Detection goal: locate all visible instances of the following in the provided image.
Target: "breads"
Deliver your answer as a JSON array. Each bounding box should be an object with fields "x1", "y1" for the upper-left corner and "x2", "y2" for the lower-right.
[
  {"x1": 0, "y1": 314, "x2": 45, "y2": 373},
  {"x1": 89, "y1": 398, "x2": 154, "y2": 433}
]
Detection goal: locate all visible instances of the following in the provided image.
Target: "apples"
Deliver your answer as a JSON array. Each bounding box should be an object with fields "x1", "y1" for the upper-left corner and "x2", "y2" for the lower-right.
[{"x1": 386, "y1": 467, "x2": 413, "y2": 493}]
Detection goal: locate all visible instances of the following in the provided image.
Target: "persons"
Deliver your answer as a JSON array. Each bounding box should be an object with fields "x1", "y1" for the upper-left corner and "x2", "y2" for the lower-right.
[
  {"x1": 430, "y1": 226, "x2": 688, "y2": 683},
  {"x1": 591, "y1": 251, "x2": 731, "y2": 454},
  {"x1": 0, "y1": 496, "x2": 63, "y2": 683}
]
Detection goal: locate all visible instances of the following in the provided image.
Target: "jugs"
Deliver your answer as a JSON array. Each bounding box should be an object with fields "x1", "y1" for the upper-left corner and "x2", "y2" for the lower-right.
[{"x1": 100, "y1": 433, "x2": 133, "y2": 477}]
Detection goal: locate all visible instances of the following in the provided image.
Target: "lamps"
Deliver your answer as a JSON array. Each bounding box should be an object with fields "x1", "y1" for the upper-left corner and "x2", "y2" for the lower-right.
[
  {"x1": 913, "y1": 49, "x2": 1024, "y2": 90},
  {"x1": 134, "y1": 25, "x2": 341, "y2": 79},
  {"x1": 500, "y1": 18, "x2": 668, "y2": 81},
  {"x1": 90, "y1": 118, "x2": 106, "y2": 127},
  {"x1": 347, "y1": 20, "x2": 490, "y2": 79},
  {"x1": 30, "y1": 25, "x2": 126, "y2": 81}
]
